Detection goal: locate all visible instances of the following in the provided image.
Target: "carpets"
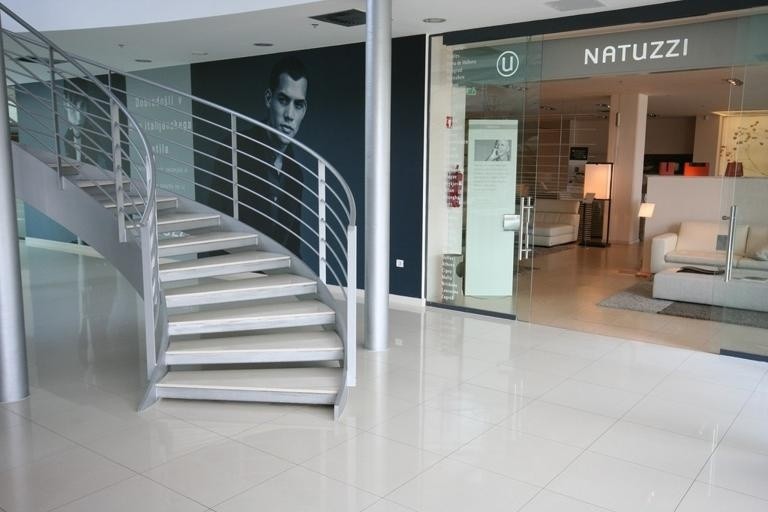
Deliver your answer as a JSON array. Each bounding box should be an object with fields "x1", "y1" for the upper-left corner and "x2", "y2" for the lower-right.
[
  {"x1": 515, "y1": 245, "x2": 573, "y2": 260},
  {"x1": 597, "y1": 280, "x2": 767, "y2": 330}
]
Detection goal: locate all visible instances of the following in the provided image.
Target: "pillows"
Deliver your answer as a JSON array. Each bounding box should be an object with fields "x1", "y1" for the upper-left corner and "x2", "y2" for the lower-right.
[
  {"x1": 676, "y1": 221, "x2": 749, "y2": 256},
  {"x1": 745, "y1": 226, "x2": 768, "y2": 256}
]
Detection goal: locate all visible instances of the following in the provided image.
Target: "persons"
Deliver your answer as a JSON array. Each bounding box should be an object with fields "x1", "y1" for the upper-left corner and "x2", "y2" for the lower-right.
[
  {"x1": 62, "y1": 74, "x2": 104, "y2": 167},
  {"x1": 203, "y1": 62, "x2": 313, "y2": 270},
  {"x1": 568, "y1": 166, "x2": 580, "y2": 184},
  {"x1": 485, "y1": 139, "x2": 510, "y2": 162}
]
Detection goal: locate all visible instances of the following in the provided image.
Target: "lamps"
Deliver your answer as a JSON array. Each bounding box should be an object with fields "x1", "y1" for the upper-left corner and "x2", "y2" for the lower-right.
[{"x1": 582, "y1": 161, "x2": 613, "y2": 247}]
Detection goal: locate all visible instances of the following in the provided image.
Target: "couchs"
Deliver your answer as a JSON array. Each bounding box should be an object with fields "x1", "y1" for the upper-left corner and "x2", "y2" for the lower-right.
[
  {"x1": 650, "y1": 232, "x2": 768, "y2": 274},
  {"x1": 515, "y1": 199, "x2": 580, "y2": 247}
]
